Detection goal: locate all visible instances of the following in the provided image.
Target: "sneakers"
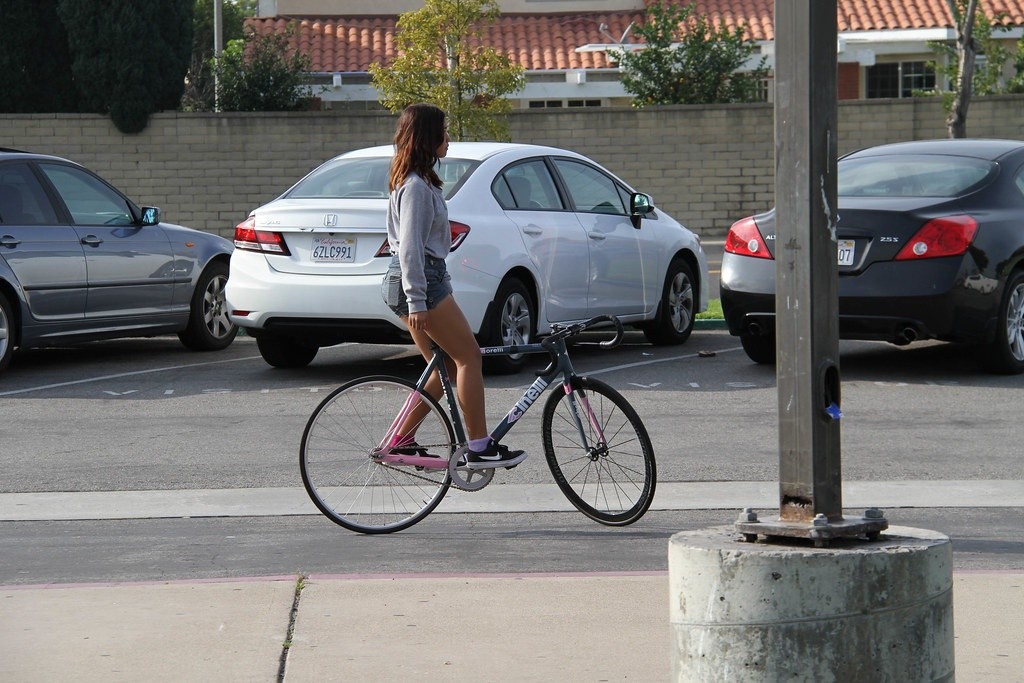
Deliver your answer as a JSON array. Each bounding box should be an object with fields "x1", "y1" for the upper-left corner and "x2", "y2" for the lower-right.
[
  {"x1": 380, "y1": 441, "x2": 443, "y2": 465},
  {"x1": 466, "y1": 438, "x2": 528, "y2": 468}
]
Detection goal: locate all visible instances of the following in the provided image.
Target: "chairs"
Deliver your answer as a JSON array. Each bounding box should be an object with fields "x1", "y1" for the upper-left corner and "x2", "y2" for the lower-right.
[
  {"x1": 498, "y1": 176, "x2": 539, "y2": 210},
  {"x1": 0, "y1": 184, "x2": 37, "y2": 224}
]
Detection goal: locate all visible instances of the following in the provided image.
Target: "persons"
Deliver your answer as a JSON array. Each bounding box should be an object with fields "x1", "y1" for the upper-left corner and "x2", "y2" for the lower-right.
[{"x1": 379, "y1": 103, "x2": 527, "y2": 469}]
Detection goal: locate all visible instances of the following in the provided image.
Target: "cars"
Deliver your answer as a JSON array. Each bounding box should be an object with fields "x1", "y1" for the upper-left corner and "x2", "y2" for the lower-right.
[
  {"x1": 719, "y1": 139, "x2": 1024, "y2": 374},
  {"x1": 225, "y1": 141, "x2": 708, "y2": 368},
  {"x1": 0, "y1": 147, "x2": 240, "y2": 376}
]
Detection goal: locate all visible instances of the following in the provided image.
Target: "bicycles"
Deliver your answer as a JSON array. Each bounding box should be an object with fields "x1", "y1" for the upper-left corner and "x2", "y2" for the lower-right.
[{"x1": 299, "y1": 314, "x2": 657, "y2": 534}]
